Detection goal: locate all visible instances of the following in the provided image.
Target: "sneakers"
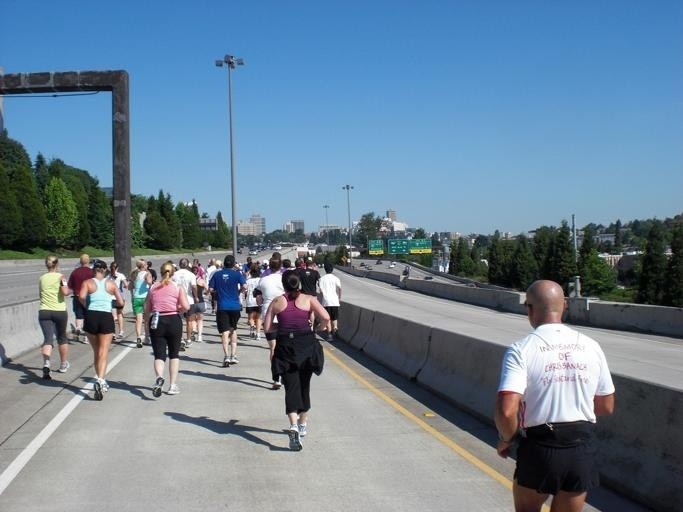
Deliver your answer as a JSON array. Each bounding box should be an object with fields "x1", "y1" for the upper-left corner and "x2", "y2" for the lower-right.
[
  {"x1": 59, "y1": 360, "x2": 69, "y2": 373},
  {"x1": 180, "y1": 330, "x2": 202, "y2": 350},
  {"x1": 249, "y1": 324, "x2": 256, "y2": 337},
  {"x1": 223, "y1": 356, "x2": 230, "y2": 367},
  {"x1": 297, "y1": 419, "x2": 307, "y2": 437},
  {"x1": 230, "y1": 354, "x2": 240, "y2": 363},
  {"x1": 255, "y1": 334, "x2": 261, "y2": 341},
  {"x1": 74, "y1": 325, "x2": 81, "y2": 341},
  {"x1": 272, "y1": 376, "x2": 281, "y2": 390},
  {"x1": 93, "y1": 379, "x2": 110, "y2": 400},
  {"x1": 167, "y1": 385, "x2": 180, "y2": 395},
  {"x1": 152, "y1": 376, "x2": 164, "y2": 398},
  {"x1": 42, "y1": 360, "x2": 52, "y2": 380},
  {"x1": 137, "y1": 336, "x2": 142, "y2": 348},
  {"x1": 119, "y1": 330, "x2": 125, "y2": 338},
  {"x1": 288, "y1": 425, "x2": 302, "y2": 452}
]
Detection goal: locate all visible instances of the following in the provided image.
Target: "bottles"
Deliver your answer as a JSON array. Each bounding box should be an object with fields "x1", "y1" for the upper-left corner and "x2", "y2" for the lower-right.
[{"x1": 149, "y1": 311, "x2": 160, "y2": 329}]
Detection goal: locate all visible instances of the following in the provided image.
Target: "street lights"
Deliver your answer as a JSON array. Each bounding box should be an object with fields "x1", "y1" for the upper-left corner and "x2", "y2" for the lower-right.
[
  {"x1": 341, "y1": 183, "x2": 353, "y2": 267},
  {"x1": 213, "y1": 53, "x2": 245, "y2": 262},
  {"x1": 402, "y1": 266, "x2": 409, "y2": 279},
  {"x1": 321, "y1": 204, "x2": 331, "y2": 252}
]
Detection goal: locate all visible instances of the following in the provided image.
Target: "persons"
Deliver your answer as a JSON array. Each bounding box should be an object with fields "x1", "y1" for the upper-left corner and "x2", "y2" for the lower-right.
[
  {"x1": 263, "y1": 271, "x2": 330, "y2": 451},
  {"x1": 129, "y1": 252, "x2": 340, "y2": 401},
  {"x1": 494, "y1": 280, "x2": 615, "y2": 511},
  {"x1": 38, "y1": 255, "x2": 70, "y2": 379},
  {"x1": 70, "y1": 252, "x2": 129, "y2": 401}
]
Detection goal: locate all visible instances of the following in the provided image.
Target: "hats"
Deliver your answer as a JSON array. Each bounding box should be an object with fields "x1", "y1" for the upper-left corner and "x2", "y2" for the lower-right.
[
  {"x1": 94, "y1": 260, "x2": 108, "y2": 269},
  {"x1": 224, "y1": 254, "x2": 236, "y2": 266}
]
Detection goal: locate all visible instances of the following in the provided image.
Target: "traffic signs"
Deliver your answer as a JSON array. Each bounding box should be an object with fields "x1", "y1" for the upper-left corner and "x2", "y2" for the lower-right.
[
  {"x1": 387, "y1": 239, "x2": 409, "y2": 255},
  {"x1": 409, "y1": 239, "x2": 433, "y2": 254},
  {"x1": 368, "y1": 239, "x2": 383, "y2": 255}
]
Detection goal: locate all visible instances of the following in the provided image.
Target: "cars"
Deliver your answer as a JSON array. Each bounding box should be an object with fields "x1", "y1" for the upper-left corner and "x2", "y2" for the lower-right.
[
  {"x1": 236, "y1": 244, "x2": 282, "y2": 255},
  {"x1": 359, "y1": 259, "x2": 397, "y2": 271}
]
